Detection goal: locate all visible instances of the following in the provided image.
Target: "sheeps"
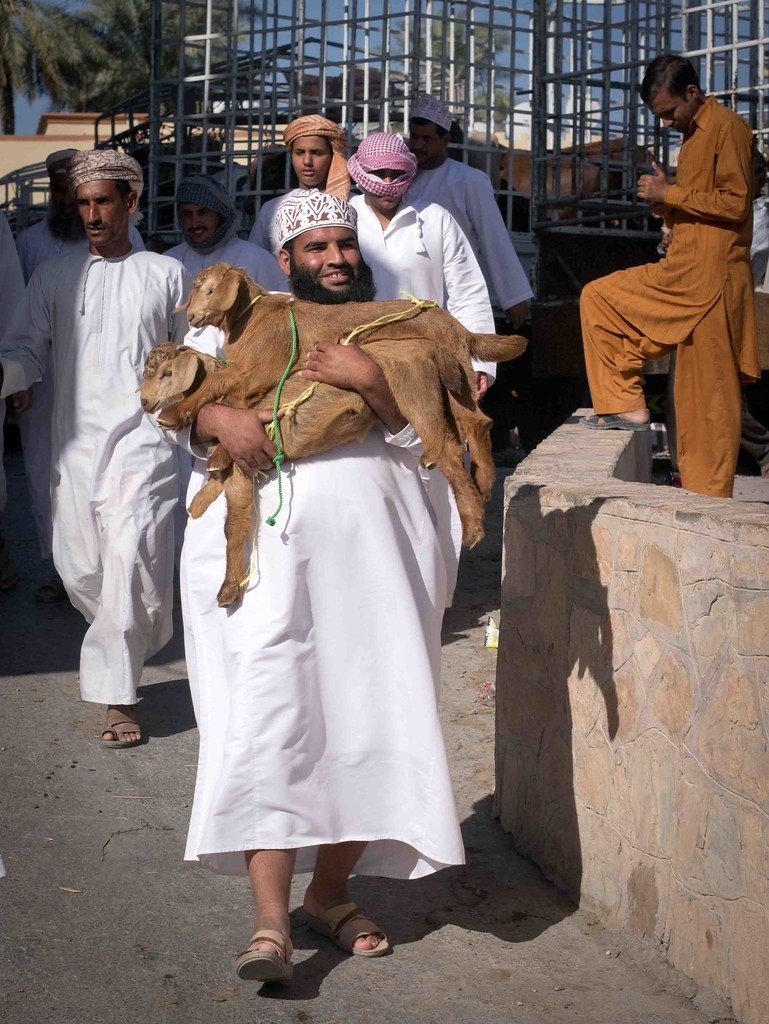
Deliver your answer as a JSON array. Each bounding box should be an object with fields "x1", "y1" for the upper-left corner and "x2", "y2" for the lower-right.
[{"x1": 133, "y1": 262, "x2": 527, "y2": 608}]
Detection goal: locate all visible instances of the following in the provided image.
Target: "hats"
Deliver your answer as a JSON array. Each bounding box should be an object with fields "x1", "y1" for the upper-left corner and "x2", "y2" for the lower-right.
[
  {"x1": 410, "y1": 94, "x2": 452, "y2": 133},
  {"x1": 268, "y1": 188, "x2": 358, "y2": 258},
  {"x1": 347, "y1": 132, "x2": 416, "y2": 197},
  {"x1": 174, "y1": 171, "x2": 249, "y2": 256},
  {"x1": 68, "y1": 148, "x2": 144, "y2": 196},
  {"x1": 46, "y1": 149, "x2": 79, "y2": 175},
  {"x1": 282, "y1": 114, "x2": 350, "y2": 201}
]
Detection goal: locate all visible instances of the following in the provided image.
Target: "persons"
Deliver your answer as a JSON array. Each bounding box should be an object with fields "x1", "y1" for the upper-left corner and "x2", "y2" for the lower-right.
[
  {"x1": 579, "y1": 55, "x2": 769, "y2": 498},
  {"x1": 0, "y1": 95, "x2": 535, "y2": 747},
  {"x1": 148, "y1": 189, "x2": 463, "y2": 980}
]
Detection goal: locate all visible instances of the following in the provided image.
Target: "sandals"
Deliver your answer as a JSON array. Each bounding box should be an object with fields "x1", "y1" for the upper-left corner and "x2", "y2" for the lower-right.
[
  {"x1": 321, "y1": 901, "x2": 389, "y2": 958},
  {"x1": 100, "y1": 706, "x2": 143, "y2": 750},
  {"x1": 579, "y1": 413, "x2": 650, "y2": 432},
  {"x1": 236, "y1": 929, "x2": 294, "y2": 979}
]
